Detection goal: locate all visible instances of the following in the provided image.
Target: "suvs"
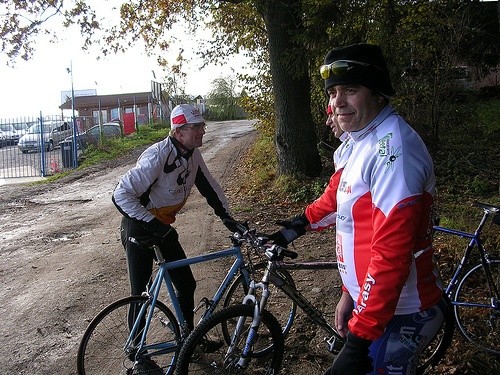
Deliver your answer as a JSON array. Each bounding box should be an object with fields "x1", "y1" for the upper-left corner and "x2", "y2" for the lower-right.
[{"x1": 108, "y1": 114, "x2": 150, "y2": 127}]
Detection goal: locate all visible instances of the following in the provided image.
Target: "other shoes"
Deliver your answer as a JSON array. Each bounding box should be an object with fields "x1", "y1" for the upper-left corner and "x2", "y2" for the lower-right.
[
  {"x1": 178, "y1": 337, "x2": 225, "y2": 352},
  {"x1": 136, "y1": 357, "x2": 165, "y2": 375}
]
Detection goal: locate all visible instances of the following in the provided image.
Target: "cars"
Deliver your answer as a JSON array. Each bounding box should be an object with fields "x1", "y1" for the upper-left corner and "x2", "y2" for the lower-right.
[
  {"x1": 64, "y1": 122, "x2": 120, "y2": 150},
  {"x1": 18, "y1": 121, "x2": 72, "y2": 154},
  {"x1": 0, "y1": 120, "x2": 37, "y2": 148}
]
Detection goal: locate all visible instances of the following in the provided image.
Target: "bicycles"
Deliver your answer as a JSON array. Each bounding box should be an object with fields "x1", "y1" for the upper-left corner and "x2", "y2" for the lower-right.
[
  {"x1": 173, "y1": 235, "x2": 457, "y2": 375},
  {"x1": 76, "y1": 221, "x2": 299, "y2": 375},
  {"x1": 431, "y1": 199, "x2": 500, "y2": 356}
]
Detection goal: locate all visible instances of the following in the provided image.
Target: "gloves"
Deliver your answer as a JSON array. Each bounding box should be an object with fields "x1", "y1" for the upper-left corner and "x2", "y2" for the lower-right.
[
  {"x1": 221, "y1": 216, "x2": 249, "y2": 235},
  {"x1": 157, "y1": 226, "x2": 180, "y2": 244},
  {"x1": 265, "y1": 215, "x2": 307, "y2": 244},
  {"x1": 320, "y1": 335, "x2": 373, "y2": 375}
]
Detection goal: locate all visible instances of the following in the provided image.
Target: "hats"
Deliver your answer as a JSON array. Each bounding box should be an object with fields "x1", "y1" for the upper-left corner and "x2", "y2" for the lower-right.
[
  {"x1": 323, "y1": 43, "x2": 396, "y2": 97},
  {"x1": 170, "y1": 104, "x2": 205, "y2": 130}
]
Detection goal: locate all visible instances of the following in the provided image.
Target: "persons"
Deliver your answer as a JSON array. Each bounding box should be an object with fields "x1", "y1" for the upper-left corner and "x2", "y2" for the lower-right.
[
  {"x1": 259, "y1": 44, "x2": 448, "y2": 375},
  {"x1": 111, "y1": 104, "x2": 250, "y2": 375}
]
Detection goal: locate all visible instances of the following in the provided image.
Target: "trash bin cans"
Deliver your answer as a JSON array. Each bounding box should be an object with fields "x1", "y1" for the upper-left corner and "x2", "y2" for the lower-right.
[{"x1": 59, "y1": 140, "x2": 77, "y2": 168}]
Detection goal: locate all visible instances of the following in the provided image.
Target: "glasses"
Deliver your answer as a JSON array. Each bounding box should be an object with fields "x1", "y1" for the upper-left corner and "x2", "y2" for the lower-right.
[
  {"x1": 318, "y1": 59, "x2": 371, "y2": 79},
  {"x1": 179, "y1": 123, "x2": 207, "y2": 132}
]
